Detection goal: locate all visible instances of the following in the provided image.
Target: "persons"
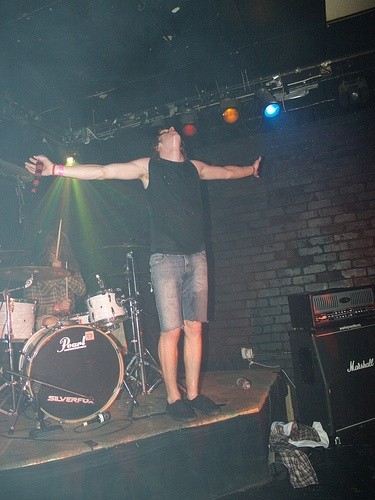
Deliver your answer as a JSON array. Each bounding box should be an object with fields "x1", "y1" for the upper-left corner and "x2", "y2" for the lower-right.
[
  {"x1": 29, "y1": 238, "x2": 87, "y2": 331},
  {"x1": 25, "y1": 124, "x2": 264, "y2": 422}
]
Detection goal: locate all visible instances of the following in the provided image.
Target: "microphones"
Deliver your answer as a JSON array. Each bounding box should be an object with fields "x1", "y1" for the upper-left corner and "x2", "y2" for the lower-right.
[{"x1": 30, "y1": 159, "x2": 43, "y2": 196}]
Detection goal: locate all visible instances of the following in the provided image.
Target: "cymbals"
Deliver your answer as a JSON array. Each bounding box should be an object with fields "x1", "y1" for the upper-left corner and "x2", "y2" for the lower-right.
[
  {"x1": 101, "y1": 240, "x2": 148, "y2": 249},
  {"x1": 1, "y1": 266, "x2": 73, "y2": 282}
]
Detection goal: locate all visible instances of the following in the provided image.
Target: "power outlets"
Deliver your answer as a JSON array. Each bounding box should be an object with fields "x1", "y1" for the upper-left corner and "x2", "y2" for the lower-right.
[{"x1": 242, "y1": 347, "x2": 253, "y2": 359}]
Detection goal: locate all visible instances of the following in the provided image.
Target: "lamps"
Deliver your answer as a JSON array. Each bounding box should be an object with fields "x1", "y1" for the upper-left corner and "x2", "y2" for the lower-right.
[
  {"x1": 254, "y1": 86, "x2": 283, "y2": 119},
  {"x1": 339, "y1": 76, "x2": 370, "y2": 107},
  {"x1": 220, "y1": 95, "x2": 241, "y2": 126},
  {"x1": 180, "y1": 110, "x2": 201, "y2": 137}
]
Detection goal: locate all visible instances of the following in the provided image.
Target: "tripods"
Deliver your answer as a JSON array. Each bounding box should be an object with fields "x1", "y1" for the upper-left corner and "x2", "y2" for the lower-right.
[{"x1": 103, "y1": 237, "x2": 187, "y2": 420}]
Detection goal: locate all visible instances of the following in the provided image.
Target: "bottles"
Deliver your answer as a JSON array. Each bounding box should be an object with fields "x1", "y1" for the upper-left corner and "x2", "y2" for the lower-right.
[{"x1": 236, "y1": 378, "x2": 251, "y2": 392}]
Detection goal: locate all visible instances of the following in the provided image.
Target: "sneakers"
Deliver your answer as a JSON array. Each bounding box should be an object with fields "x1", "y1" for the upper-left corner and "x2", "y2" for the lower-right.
[
  {"x1": 165, "y1": 398, "x2": 196, "y2": 422},
  {"x1": 184, "y1": 394, "x2": 222, "y2": 416}
]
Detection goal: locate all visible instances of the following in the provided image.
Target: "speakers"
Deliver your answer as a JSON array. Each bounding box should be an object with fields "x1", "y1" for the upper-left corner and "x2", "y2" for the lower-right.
[{"x1": 289, "y1": 315, "x2": 375, "y2": 446}]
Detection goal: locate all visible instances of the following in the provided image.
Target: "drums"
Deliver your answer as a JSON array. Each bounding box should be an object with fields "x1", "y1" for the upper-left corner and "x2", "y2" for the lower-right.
[
  {"x1": 85, "y1": 286, "x2": 128, "y2": 326},
  {"x1": 69, "y1": 311, "x2": 120, "y2": 334},
  {"x1": 0, "y1": 298, "x2": 38, "y2": 342},
  {"x1": 18, "y1": 320, "x2": 124, "y2": 423}
]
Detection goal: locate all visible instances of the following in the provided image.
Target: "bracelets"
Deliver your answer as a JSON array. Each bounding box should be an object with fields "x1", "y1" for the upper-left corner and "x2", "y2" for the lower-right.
[
  {"x1": 54, "y1": 303, "x2": 59, "y2": 312},
  {"x1": 50, "y1": 164, "x2": 65, "y2": 177}
]
